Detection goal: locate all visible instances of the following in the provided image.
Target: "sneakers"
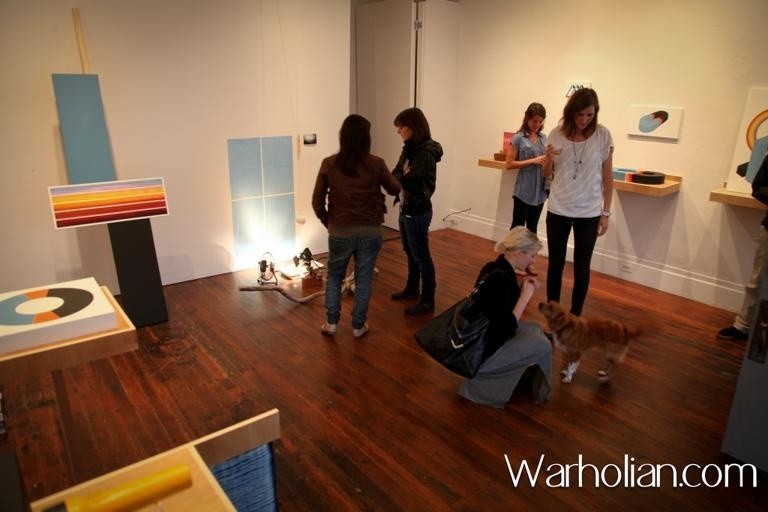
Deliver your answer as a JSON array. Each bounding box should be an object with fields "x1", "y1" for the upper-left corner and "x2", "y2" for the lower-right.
[
  {"x1": 391, "y1": 288, "x2": 420, "y2": 301},
  {"x1": 320, "y1": 322, "x2": 337, "y2": 335},
  {"x1": 406, "y1": 303, "x2": 434, "y2": 316},
  {"x1": 716, "y1": 326, "x2": 748, "y2": 340},
  {"x1": 352, "y1": 323, "x2": 369, "y2": 339}
]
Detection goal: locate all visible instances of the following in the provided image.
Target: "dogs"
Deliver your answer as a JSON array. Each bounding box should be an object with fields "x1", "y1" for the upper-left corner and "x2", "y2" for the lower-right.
[{"x1": 538, "y1": 301, "x2": 643, "y2": 383}]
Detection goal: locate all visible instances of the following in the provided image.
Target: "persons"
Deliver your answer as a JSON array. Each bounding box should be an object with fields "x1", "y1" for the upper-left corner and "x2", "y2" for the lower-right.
[
  {"x1": 540, "y1": 88, "x2": 614, "y2": 342},
  {"x1": 387, "y1": 107, "x2": 443, "y2": 316},
  {"x1": 506, "y1": 102, "x2": 555, "y2": 234},
  {"x1": 312, "y1": 114, "x2": 402, "y2": 339},
  {"x1": 456, "y1": 225, "x2": 553, "y2": 410},
  {"x1": 717, "y1": 154, "x2": 768, "y2": 341}
]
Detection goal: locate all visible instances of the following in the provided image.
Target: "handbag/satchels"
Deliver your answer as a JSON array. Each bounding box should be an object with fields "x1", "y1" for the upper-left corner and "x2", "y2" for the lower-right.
[
  {"x1": 544, "y1": 173, "x2": 553, "y2": 193},
  {"x1": 413, "y1": 295, "x2": 491, "y2": 380}
]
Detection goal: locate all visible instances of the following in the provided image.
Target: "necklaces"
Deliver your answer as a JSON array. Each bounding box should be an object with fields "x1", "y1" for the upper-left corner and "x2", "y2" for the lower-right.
[{"x1": 572, "y1": 135, "x2": 588, "y2": 180}]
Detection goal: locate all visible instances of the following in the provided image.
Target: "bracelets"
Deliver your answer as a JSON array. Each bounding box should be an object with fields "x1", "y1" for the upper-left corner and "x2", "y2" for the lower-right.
[{"x1": 600, "y1": 211, "x2": 611, "y2": 216}]
